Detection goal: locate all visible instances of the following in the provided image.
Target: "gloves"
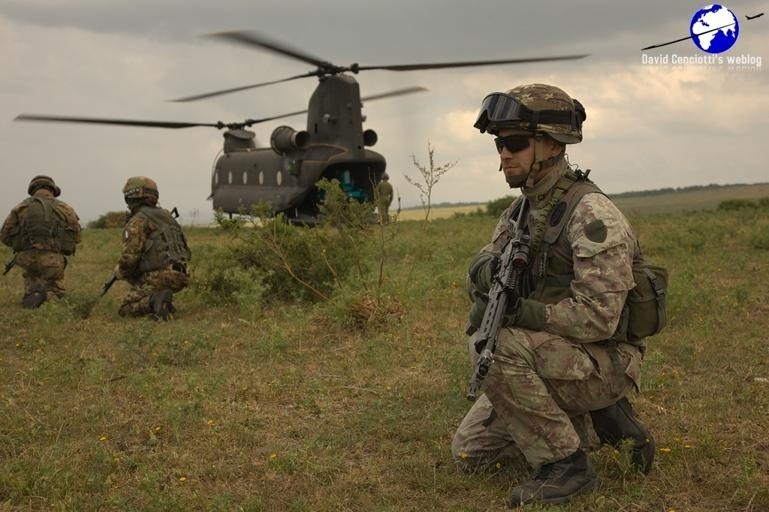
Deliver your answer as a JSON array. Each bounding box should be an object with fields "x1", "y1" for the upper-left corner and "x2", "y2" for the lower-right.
[{"x1": 466, "y1": 290, "x2": 545, "y2": 332}]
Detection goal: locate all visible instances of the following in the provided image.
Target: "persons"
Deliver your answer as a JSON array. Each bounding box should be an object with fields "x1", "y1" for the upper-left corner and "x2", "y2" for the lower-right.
[
  {"x1": 452, "y1": 84, "x2": 656, "y2": 508},
  {"x1": 0, "y1": 175, "x2": 82, "y2": 310},
  {"x1": 115, "y1": 177, "x2": 190, "y2": 320}
]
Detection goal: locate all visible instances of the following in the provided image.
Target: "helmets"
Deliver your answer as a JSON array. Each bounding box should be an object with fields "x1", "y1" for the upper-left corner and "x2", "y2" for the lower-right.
[
  {"x1": 28, "y1": 176, "x2": 60, "y2": 196},
  {"x1": 123, "y1": 176, "x2": 158, "y2": 204},
  {"x1": 478, "y1": 83, "x2": 586, "y2": 144}
]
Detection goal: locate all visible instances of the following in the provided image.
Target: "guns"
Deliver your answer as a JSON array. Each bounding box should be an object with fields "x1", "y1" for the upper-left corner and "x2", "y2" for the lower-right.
[
  {"x1": 3, "y1": 256, "x2": 16, "y2": 276},
  {"x1": 466, "y1": 197, "x2": 532, "y2": 400},
  {"x1": 98, "y1": 208, "x2": 179, "y2": 296}
]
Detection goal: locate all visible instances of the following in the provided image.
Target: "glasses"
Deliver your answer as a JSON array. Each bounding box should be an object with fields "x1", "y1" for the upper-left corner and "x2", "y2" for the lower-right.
[
  {"x1": 474, "y1": 92, "x2": 537, "y2": 128},
  {"x1": 496, "y1": 134, "x2": 535, "y2": 154}
]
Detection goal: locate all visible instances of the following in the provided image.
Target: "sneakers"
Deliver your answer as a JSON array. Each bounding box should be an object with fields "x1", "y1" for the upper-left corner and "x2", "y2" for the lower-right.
[
  {"x1": 512, "y1": 450, "x2": 598, "y2": 505},
  {"x1": 153, "y1": 289, "x2": 173, "y2": 321},
  {"x1": 23, "y1": 292, "x2": 46, "y2": 309},
  {"x1": 593, "y1": 398, "x2": 655, "y2": 478}
]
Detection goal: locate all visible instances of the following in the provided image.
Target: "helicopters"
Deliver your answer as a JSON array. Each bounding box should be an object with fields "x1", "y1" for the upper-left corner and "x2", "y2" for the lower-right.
[{"x1": 11, "y1": 30, "x2": 592, "y2": 230}]
